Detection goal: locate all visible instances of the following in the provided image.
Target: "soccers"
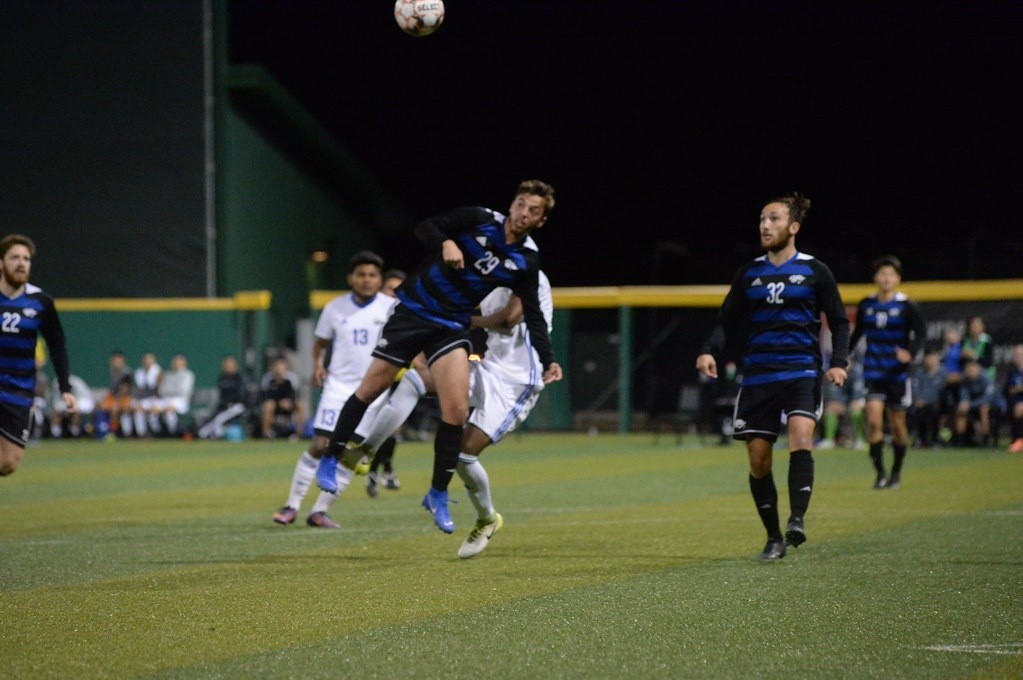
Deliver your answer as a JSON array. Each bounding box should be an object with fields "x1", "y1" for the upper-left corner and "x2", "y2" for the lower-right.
[{"x1": 394, "y1": 0, "x2": 445, "y2": 36}]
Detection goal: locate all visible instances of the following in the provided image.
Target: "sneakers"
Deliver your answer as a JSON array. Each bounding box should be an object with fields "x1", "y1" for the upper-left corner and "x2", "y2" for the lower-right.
[
  {"x1": 786, "y1": 517, "x2": 806, "y2": 547},
  {"x1": 307, "y1": 513, "x2": 343, "y2": 528},
  {"x1": 458, "y1": 512, "x2": 503, "y2": 560},
  {"x1": 272, "y1": 505, "x2": 296, "y2": 525},
  {"x1": 760, "y1": 540, "x2": 786, "y2": 560},
  {"x1": 340, "y1": 442, "x2": 370, "y2": 476},
  {"x1": 420, "y1": 487, "x2": 455, "y2": 535},
  {"x1": 316, "y1": 455, "x2": 337, "y2": 494}
]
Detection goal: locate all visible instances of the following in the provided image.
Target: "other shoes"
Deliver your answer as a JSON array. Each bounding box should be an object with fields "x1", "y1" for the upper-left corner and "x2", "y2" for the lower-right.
[
  {"x1": 887, "y1": 477, "x2": 901, "y2": 489},
  {"x1": 376, "y1": 471, "x2": 400, "y2": 489},
  {"x1": 365, "y1": 472, "x2": 377, "y2": 497},
  {"x1": 1009, "y1": 438, "x2": 1023, "y2": 452},
  {"x1": 874, "y1": 477, "x2": 886, "y2": 487}
]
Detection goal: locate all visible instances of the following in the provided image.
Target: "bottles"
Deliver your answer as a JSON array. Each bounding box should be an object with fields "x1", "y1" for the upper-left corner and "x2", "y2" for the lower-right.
[{"x1": 726, "y1": 361, "x2": 737, "y2": 383}]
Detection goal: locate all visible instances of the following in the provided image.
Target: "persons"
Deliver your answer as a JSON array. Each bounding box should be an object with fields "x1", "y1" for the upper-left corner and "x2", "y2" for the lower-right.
[
  {"x1": 1002, "y1": 344, "x2": 1023, "y2": 453},
  {"x1": 261, "y1": 356, "x2": 304, "y2": 440},
  {"x1": 696, "y1": 198, "x2": 848, "y2": 560},
  {"x1": 316, "y1": 180, "x2": 562, "y2": 535},
  {"x1": 848, "y1": 257, "x2": 921, "y2": 490},
  {"x1": 218, "y1": 356, "x2": 244, "y2": 411},
  {"x1": 911, "y1": 315, "x2": 997, "y2": 449},
  {"x1": 28, "y1": 373, "x2": 48, "y2": 441},
  {"x1": 106, "y1": 350, "x2": 194, "y2": 436},
  {"x1": 368, "y1": 268, "x2": 406, "y2": 499},
  {"x1": 272, "y1": 262, "x2": 395, "y2": 528},
  {"x1": 337, "y1": 269, "x2": 553, "y2": 559},
  {"x1": 818, "y1": 323, "x2": 866, "y2": 449},
  {"x1": 49, "y1": 374, "x2": 96, "y2": 436},
  {"x1": 0, "y1": 234, "x2": 76, "y2": 477}
]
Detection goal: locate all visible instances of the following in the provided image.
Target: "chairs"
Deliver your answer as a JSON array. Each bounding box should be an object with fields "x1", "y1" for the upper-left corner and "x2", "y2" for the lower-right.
[{"x1": 189, "y1": 388, "x2": 219, "y2": 432}]
